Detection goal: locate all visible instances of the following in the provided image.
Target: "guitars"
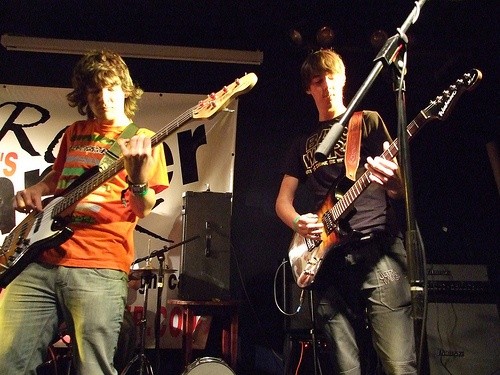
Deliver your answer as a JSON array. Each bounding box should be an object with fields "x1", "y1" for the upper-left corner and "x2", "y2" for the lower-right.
[
  {"x1": 287, "y1": 67, "x2": 483, "y2": 290},
  {"x1": 0, "y1": 72, "x2": 259, "y2": 287}
]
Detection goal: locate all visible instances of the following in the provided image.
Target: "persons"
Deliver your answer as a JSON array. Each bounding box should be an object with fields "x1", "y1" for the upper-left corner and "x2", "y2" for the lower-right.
[
  {"x1": 0, "y1": 50, "x2": 169, "y2": 375},
  {"x1": 275, "y1": 50, "x2": 417, "y2": 375}
]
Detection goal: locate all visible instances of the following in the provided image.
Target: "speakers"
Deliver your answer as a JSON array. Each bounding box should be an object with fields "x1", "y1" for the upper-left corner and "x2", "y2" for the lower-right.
[
  {"x1": 419, "y1": 294, "x2": 500, "y2": 375},
  {"x1": 281, "y1": 257, "x2": 319, "y2": 333}
]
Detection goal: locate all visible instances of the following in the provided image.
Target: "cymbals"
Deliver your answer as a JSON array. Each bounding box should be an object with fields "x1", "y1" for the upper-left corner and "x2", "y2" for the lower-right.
[{"x1": 129, "y1": 268, "x2": 178, "y2": 280}]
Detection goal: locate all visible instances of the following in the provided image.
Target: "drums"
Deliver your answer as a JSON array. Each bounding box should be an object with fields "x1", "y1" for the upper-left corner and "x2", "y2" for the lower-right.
[{"x1": 182, "y1": 357, "x2": 236, "y2": 375}]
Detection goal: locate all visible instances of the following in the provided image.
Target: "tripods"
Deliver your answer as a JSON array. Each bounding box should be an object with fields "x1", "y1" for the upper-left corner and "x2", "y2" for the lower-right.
[{"x1": 119, "y1": 283, "x2": 154, "y2": 375}]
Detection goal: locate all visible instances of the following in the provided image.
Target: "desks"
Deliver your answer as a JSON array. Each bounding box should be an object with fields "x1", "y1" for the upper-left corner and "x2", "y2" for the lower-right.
[{"x1": 168, "y1": 298, "x2": 250, "y2": 367}]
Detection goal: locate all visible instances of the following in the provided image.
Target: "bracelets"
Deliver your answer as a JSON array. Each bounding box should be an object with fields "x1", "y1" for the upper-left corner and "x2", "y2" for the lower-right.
[
  {"x1": 121, "y1": 181, "x2": 148, "y2": 206},
  {"x1": 293, "y1": 216, "x2": 300, "y2": 232}
]
